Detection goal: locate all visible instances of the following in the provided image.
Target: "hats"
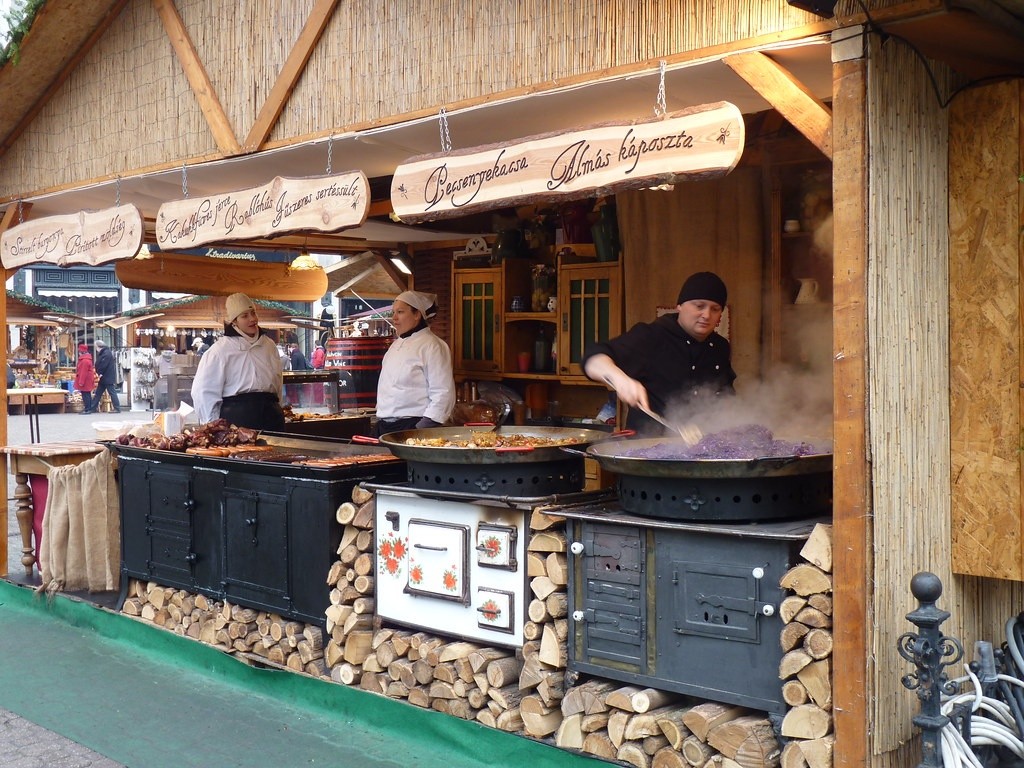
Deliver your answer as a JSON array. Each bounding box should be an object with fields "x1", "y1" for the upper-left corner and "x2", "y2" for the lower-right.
[
  {"x1": 224, "y1": 292, "x2": 256, "y2": 323},
  {"x1": 78, "y1": 344, "x2": 88, "y2": 350},
  {"x1": 675, "y1": 271, "x2": 728, "y2": 308},
  {"x1": 192, "y1": 337, "x2": 203, "y2": 347}
]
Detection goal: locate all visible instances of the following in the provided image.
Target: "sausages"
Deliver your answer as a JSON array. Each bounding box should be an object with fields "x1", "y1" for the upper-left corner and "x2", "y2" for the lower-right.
[{"x1": 185, "y1": 444, "x2": 397, "y2": 466}]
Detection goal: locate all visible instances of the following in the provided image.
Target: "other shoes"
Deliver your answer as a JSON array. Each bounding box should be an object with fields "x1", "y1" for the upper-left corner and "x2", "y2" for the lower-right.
[
  {"x1": 79, "y1": 409, "x2": 96, "y2": 413},
  {"x1": 108, "y1": 409, "x2": 118, "y2": 412}
]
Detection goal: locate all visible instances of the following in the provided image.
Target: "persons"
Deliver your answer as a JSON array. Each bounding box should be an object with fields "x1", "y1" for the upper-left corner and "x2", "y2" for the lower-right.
[
  {"x1": 309, "y1": 340, "x2": 325, "y2": 406},
  {"x1": 372, "y1": 291, "x2": 456, "y2": 440},
  {"x1": 159, "y1": 344, "x2": 175, "y2": 412},
  {"x1": 73, "y1": 345, "x2": 94, "y2": 414},
  {"x1": 188, "y1": 337, "x2": 209, "y2": 356},
  {"x1": 276, "y1": 343, "x2": 306, "y2": 408},
  {"x1": 6, "y1": 363, "x2": 16, "y2": 417},
  {"x1": 580, "y1": 272, "x2": 737, "y2": 440},
  {"x1": 91, "y1": 340, "x2": 121, "y2": 414},
  {"x1": 191, "y1": 293, "x2": 285, "y2": 433}
]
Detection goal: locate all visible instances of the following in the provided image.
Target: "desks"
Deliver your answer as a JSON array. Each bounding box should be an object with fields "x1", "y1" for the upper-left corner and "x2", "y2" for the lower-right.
[
  {"x1": 0, "y1": 441, "x2": 103, "y2": 573},
  {"x1": 7, "y1": 388, "x2": 70, "y2": 415}
]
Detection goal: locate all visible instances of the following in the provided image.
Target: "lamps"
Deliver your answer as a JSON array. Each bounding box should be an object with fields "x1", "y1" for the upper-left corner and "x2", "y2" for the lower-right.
[
  {"x1": 391, "y1": 243, "x2": 416, "y2": 276},
  {"x1": 137, "y1": 241, "x2": 154, "y2": 261},
  {"x1": 289, "y1": 236, "x2": 323, "y2": 270}
]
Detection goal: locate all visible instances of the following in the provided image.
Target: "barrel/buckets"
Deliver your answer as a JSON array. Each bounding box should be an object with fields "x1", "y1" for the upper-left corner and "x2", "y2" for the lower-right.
[{"x1": 324, "y1": 337, "x2": 393, "y2": 412}]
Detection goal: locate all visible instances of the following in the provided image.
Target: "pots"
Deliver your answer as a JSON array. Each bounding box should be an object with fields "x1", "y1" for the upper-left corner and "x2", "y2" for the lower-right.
[
  {"x1": 351, "y1": 422, "x2": 635, "y2": 464},
  {"x1": 557, "y1": 435, "x2": 833, "y2": 478}
]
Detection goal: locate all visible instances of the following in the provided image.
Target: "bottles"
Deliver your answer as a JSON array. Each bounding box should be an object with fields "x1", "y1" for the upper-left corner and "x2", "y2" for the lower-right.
[
  {"x1": 530, "y1": 328, "x2": 557, "y2": 372},
  {"x1": 547, "y1": 296, "x2": 557, "y2": 313},
  {"x1": 510, "y1": 295, "x2": 527, "y2": 312},
  {"x1": 590, "y1": 204, "x2": 618, "y2": 262},
  {"x1": 492, "y1": 229, "x2": 524, "y2": 266}
]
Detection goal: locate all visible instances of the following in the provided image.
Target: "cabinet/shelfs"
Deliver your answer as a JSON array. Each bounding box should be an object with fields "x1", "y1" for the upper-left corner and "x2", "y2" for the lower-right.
[
  {"x1": 772, "y1": 166, "x2": 833, "y2": 377},
  {"x1": 558, "y1": 254, "x2": 624, "y2": 388},
  {"x1": 444, "y1": 255, "x2": 519, "y2": 377},
  {"x1": 502, "y1": 310, "x2": 560, "y2": 380}
]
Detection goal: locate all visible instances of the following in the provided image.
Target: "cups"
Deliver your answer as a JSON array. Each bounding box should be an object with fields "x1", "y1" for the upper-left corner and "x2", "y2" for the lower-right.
[{"x1": 518, "y1": 352, "x2": 529, "y2": 372}]
